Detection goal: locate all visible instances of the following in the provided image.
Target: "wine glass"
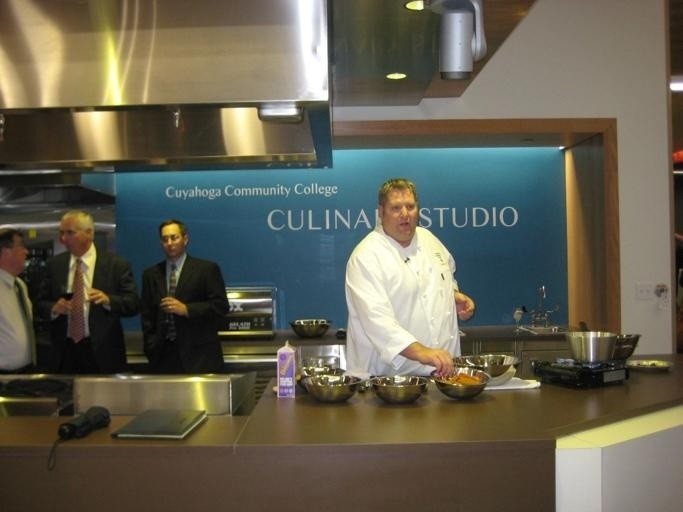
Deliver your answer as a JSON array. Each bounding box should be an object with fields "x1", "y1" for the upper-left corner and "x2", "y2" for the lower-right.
[{"x1": 63, "y1": 284, "x2": 75, "y2": 315}]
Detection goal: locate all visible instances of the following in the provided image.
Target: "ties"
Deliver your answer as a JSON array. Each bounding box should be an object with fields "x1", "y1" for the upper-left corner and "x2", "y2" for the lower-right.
[
  {"x1": 15, "y1": 281, "x2": 27, "y2": 318},
  {"x1": 71, "y1": 260, "x2": 85, "y2": 344},
  {"x1": 163, "y1": 266, "x2": 178, "y2": 342}
]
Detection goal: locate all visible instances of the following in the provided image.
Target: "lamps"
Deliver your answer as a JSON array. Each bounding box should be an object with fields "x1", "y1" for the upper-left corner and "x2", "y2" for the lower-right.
[{"x1": 437, "y1": 0, "x2": 487, "y2": 81}]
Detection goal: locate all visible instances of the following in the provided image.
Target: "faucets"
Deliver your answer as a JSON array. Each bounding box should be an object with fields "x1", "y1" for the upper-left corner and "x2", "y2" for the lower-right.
[{"x1": 535, "y1": 281, "x2": 547, "y2": 312}]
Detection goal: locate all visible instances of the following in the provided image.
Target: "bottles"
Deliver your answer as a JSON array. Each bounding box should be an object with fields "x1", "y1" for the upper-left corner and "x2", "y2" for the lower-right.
[{"x1": 276, "y1": 340, "x2": 298, "y2": 399}]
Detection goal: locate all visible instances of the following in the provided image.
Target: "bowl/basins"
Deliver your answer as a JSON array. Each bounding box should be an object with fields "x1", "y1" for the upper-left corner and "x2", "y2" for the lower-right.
[
  {"x1": 451, "y1": 353, "x2": 523, "y2": 387},
  {"x1": 613, "y1": 334, "x2": 641, "y2": 360},
  {"x1": 300, "y1": 374, "x2": 362, "y2": 403},
  {"x1": 431, "y1": 366, "x2": 491, "y2": 401},
  {"x1": 296, "y1": 366, "x2": 345, "y2": 391},
  {"x1": 288, "y1": 318, "x2": 331, "y2": 339},
  {"x1": 368, "y1": 374, "x2": 428, "y2": 405}
]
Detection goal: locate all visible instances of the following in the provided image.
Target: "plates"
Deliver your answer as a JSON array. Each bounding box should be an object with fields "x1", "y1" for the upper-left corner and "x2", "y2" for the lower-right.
[{"x1": 626, "y1": 359, "x2": 673, "y2": 372}]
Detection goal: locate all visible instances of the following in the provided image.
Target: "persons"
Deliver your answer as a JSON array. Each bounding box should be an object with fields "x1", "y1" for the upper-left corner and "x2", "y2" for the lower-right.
[
  {"x1": 137, "y1": 219, "x2": 232, "y2": 375},
  {"x1": 0, "y1": 226, "x2": 38, "y2": 374},
  {"x1": 29, "y1": 209, "x2": 141, "y2": 375},
  {"x1": 344, "y1": 177, "x2": 475, "y2": 381}
]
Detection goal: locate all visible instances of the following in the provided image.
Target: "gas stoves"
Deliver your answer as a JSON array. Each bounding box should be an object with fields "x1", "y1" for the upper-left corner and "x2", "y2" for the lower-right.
[
  {"x1": 531, "y1": 356, "x2": 630, "y2": 391},
  {"x1": 0, "y1": 378, "x2": 73, "y2": 397}
]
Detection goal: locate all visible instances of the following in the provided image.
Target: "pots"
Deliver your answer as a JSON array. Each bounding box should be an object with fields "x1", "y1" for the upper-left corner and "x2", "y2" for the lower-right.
[{"x1": 567, "y1": 332, "x2": 618, "y2": 364}]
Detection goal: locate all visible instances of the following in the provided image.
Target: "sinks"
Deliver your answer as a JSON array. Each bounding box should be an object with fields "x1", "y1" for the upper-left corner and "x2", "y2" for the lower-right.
[{"x1": 519, "y1": 326, "x2": 569, "y2": 334}]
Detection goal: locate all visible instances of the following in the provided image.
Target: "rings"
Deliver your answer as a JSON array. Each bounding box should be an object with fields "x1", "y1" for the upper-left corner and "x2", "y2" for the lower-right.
[{"x1": 168, "y1": 305, "x2": 171, "y2": 310}]
